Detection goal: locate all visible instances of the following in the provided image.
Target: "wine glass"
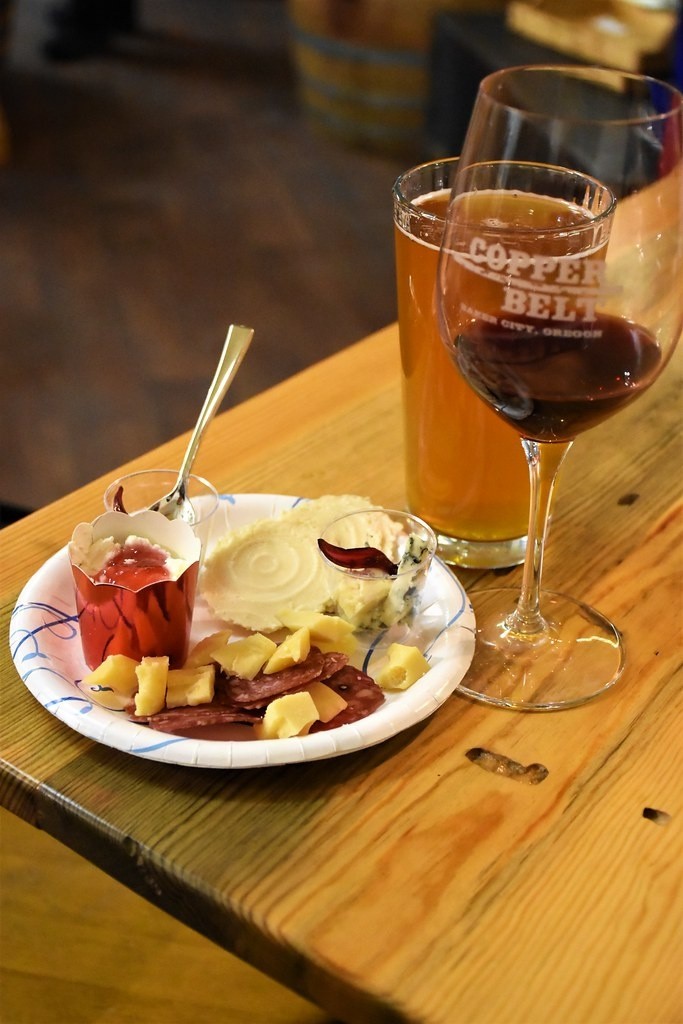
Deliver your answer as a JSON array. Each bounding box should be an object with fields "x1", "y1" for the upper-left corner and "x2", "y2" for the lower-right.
[{"x1": 444, "y1": 68, "x2": 683, "y2": 719}]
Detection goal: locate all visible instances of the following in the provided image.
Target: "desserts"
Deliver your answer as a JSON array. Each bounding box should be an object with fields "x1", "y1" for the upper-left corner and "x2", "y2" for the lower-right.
[{"x1": 68, "y1": 493, "x2": 428, "y2": 744}]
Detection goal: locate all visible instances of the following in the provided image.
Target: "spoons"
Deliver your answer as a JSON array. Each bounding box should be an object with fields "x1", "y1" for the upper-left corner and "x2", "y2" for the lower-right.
[{"x1": 146, "y1": 325, "x2": 255, "y2": 524}]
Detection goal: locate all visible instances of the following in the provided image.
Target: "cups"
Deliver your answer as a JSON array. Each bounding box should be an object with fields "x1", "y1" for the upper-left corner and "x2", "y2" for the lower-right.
[
  {"x1": 104, "y1": 468, "x2": 220, "y2": 596},
  {"x1": 393, "y1": 158, "x2": 615, "y2": 571},
  {"x1": 318, "y1": 506, "x2": 440, "y2": 649}
]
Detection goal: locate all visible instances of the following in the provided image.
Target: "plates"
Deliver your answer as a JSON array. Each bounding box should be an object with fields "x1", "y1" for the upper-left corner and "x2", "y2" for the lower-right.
[{"x1": 9, "y1": 495, "x2": 478, "y2": 771}]
